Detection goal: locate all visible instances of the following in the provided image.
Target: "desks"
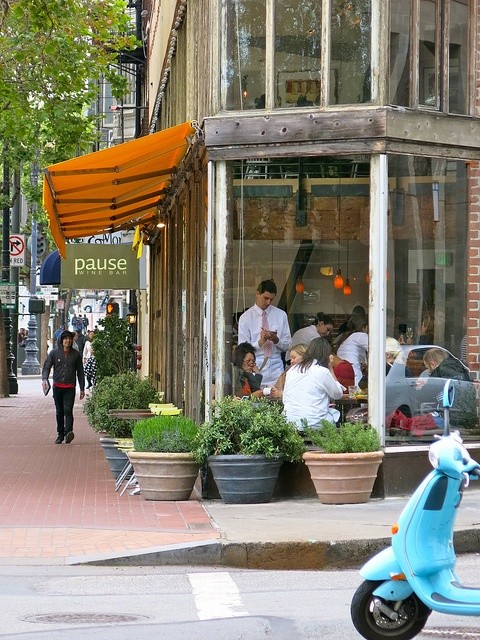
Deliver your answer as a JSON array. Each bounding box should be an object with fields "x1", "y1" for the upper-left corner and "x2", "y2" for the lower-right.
[{"x1": 330, "y1": 397, "x2": 369, "y2": 420}]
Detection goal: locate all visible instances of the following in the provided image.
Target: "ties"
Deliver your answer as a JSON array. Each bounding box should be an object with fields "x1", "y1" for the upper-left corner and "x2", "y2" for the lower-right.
[{"x1": 262, "y1": 312, "x2": 272, "y2": 358}]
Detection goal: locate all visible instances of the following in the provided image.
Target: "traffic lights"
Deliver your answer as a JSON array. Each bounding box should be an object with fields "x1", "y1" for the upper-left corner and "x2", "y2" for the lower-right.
[
  {"x1": 107, "y1": 302, "x2": 118, "y2": 314},
  {"x1": 37, "y1": 235, "x2": 44, "y2": 254}
]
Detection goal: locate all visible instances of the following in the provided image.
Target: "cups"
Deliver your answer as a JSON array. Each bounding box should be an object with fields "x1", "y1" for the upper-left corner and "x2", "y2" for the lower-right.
[{"x1": 348, "y1": 385, "x2": 357, "y2": 399}]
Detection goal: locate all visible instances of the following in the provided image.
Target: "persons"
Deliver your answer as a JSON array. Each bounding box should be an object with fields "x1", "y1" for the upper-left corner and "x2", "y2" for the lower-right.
[
  {"x1": 359, "y1": 337, "x2": 416, "y2": 427},
  {"x1": 82, "y1": 331, "x2": 99, "y2": 388},
  {"x1": 231, "y1": 342, "x2": 263, "y2": 403},
  {"x1": 337, "y1": 316, "x2": 367, "y2": 408},
  {"x1": 42, "y1": 331, "x2": 85, "y2": 444},
  {"x1": 281, "y1": 337, "x2": 343, "y2": 433},
  {"x1": 269, "y1": 343, "x2": 310, "y2": 400},
  {"x1": 71, "y1": 331, "x2": 79, "y2": 352},
  {"x1": 238, "y1": 280, "x2": 292, "y2": 395},
  {"x1": 284, "y1": 310, "x2": 334, "y2": 371},
  {"x1": 333, "y1": 305, "x2": 366, "y2": 349},
  {"x1": 416, "y1": 349, "x2": 477, "y2": 429},
  {"x1": 338, "y1": 314, "x2": 350, "y2": 333},
  {"x1": 18, "y1": 328, "x2": 27, "y2": 344},
  {"x1": 328, "y1": 350, "x2": 354, "y2": 394},
  {"x1": 415, "y1": 313, "x2": 436, "y2": 359},
  {"x1": 231, "y1": 346, "x2": 262, "y2": 390}
]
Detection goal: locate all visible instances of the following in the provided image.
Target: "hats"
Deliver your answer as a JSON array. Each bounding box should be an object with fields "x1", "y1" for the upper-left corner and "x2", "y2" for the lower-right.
[{"x1": 62, "y1": 331, "x2": 73, "y2": 337}]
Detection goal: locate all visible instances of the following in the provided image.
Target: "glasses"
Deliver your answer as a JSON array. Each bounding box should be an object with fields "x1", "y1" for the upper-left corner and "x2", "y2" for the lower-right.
[{"x1": 243, "y1": 360, "x2": 257, "y2": 366}]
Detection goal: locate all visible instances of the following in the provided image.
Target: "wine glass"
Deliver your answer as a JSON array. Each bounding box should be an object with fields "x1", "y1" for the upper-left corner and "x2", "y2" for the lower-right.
[{"x1": 406, "y1": 327, "x2": 414, "y2": 339}]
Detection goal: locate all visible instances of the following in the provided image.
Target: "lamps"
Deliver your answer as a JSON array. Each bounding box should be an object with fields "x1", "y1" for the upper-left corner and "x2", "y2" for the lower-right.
[
  {"x1": 333, "y1": 192, "x2": 345, "y2": 289},
  {"x1": 343, "y1": 238, "x2": 353, "y2": 296},
  {"x1": 321, "y1": 264, "x2": 335, "y2": 278},
  {"x1": 294, "y1": 275, "x2": 306, "y2": 295}
]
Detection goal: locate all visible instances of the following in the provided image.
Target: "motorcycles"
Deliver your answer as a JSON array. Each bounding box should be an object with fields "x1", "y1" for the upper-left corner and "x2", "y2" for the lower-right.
[{"x1": 350, "y1": 379, "x2": 479, "y2": 640}]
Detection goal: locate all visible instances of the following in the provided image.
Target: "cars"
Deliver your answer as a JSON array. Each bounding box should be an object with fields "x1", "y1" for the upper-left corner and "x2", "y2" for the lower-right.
[{"x1": 385, "y1": 345, "x2": 477, "y2": 436}]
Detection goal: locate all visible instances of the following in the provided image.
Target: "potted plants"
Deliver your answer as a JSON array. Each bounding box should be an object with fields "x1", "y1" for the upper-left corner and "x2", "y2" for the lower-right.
[
  {"x1": 300, "y1": 416, "x2": 387, "y2": 503},
  {"x1": 199, "y1": 397, "x2": 300, "y2": 505},
  {"x1": 83, "y1": 371, "x2": 162, "y2": 480},
  {"x1": 129, "y1": 413, "x2": 199, "y2": 501}
]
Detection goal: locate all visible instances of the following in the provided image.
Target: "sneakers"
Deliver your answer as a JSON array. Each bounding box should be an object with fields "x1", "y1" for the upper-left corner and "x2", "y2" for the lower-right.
[
  {"x1": 65, "y1": 431, "x2": 74, "y2": 444},
  {"x1": 55, "y1": 434, "x2": 64, "y2": 444},
  {"x1": 86, "y1": 383, "x2": 92, "y2": 389}
]
